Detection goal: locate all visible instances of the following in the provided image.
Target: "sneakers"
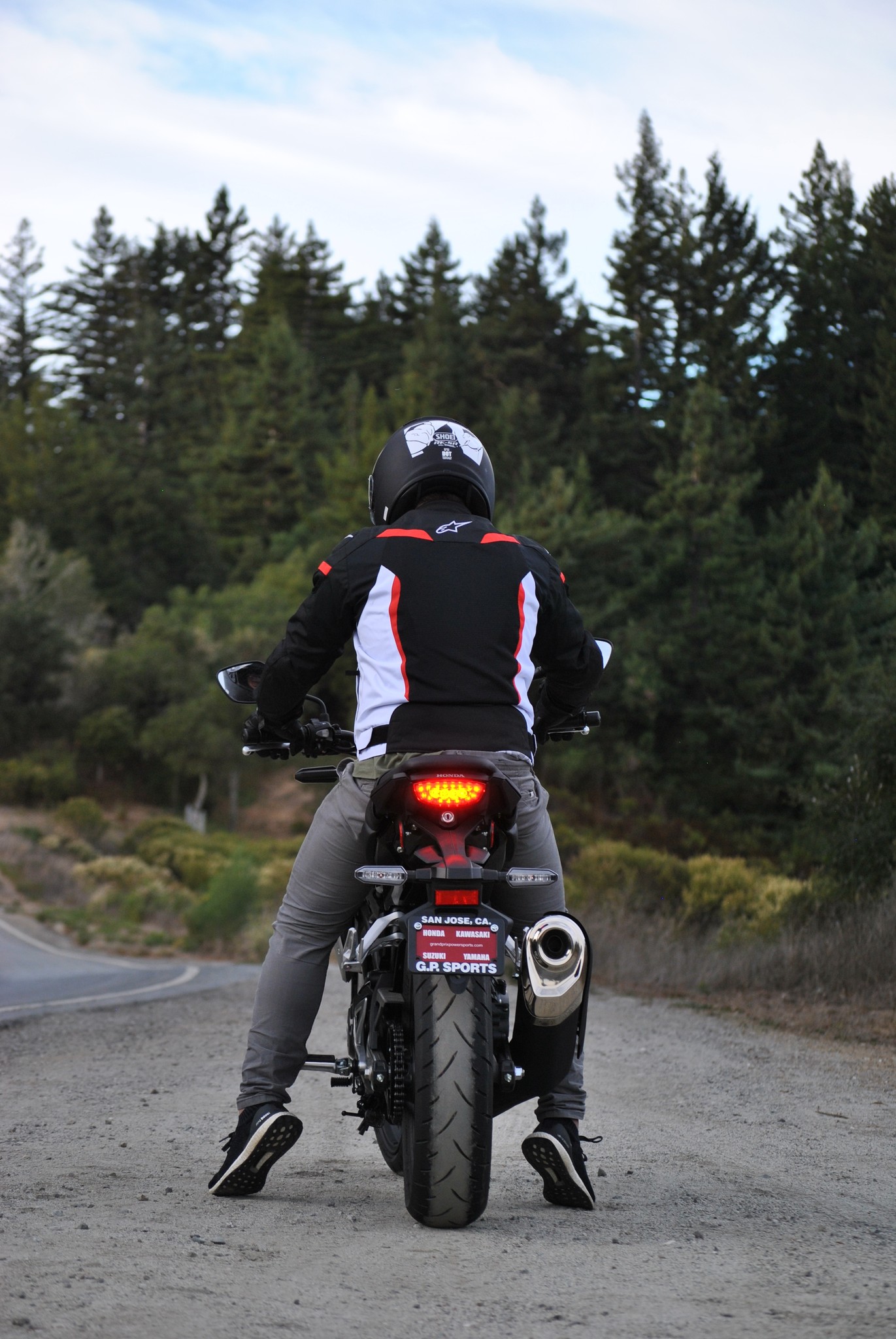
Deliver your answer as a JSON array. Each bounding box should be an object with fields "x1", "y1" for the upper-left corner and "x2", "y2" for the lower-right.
[
  {"x1": 205, "y1": 1103, "x2": 303, "y2": 1194},
  {"x1": 516, "y1": 1118, "x2": 602, "y2": 1211}
]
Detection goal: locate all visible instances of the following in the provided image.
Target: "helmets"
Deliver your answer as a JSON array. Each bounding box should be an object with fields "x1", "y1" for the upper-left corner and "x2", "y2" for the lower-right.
[
  {"x1": 369, "y1": 416, "x2": 499, "y2": 524},
  {"x1": 221, "y1": 662, "x2": 261, "y2": 701}
]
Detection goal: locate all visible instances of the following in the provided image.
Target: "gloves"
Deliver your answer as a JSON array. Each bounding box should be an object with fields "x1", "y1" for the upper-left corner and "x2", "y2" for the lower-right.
[
  {"x1": 243, "y1": 711, "x2": 306, "y2": 763},
  {"x1": 529, "y1": 691, "x2": 582, "y2": 743}
]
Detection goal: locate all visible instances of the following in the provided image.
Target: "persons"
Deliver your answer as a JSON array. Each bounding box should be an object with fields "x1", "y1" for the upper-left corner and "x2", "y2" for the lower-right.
[{"x1": 206, "y1": 422, "x2": 603, "y2": 1211}]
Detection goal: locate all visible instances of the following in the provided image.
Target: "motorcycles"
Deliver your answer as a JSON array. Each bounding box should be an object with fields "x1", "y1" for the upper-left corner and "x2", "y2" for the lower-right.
[{"x1": 217, "y1": 640, "x2": 591, "y2": 1233}]
